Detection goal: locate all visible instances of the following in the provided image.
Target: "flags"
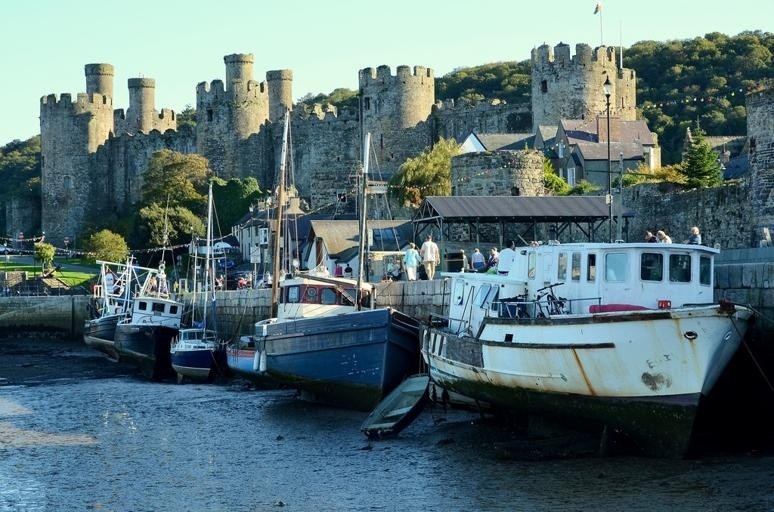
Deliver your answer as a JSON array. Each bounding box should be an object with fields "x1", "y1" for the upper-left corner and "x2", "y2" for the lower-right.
[{"x1": 594, "y1": 4, "x2": 603, "y2": 14}]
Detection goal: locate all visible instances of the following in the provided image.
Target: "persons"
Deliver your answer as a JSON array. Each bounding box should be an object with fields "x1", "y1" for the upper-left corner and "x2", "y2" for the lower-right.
[
  {"x1": 384, "y1": 234, "x2": 440, "y2": 281},
  {"x1": 643, "y1": 230, "x2": 659, "y2": 242},
  {"x1": 458, "y1": 239, "x2": 515, "y2": 276},
  {"x1": 657, "y1": 230, "x2": 673, "y2": 244},
  {"x1": 172, "y1": 278, "x2": 193, "y2": 293},
  {"x1": 529, "y1": 241, "x2": 539, "y2": 246},
  {"x1": 236, "y1": 267, "x2": 300, "y2": 288},
  {"x1": 641, "y1": 254, "x2": 652, "y2": 280},
  {"x1": 89, "y1": 281, "x2": 99, "y2": 295},
  {"x1": 334, "y1": 264, "x2": 353, "y2": 277},
  {"x1": 215, "y1": 273, "x2": 225, "y2": 291},
  {"x1": 761, "y1": 226, "x2": 773, "y2": 247},
  {"x1": 682, "y1": 226, "x2": 702, "y2": 245}
]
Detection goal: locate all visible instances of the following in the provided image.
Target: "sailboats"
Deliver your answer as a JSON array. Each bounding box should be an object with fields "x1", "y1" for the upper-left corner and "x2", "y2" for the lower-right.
[
  {"x1": 111, "y1": 191, "x2": 185, "y2": 378},
  {"x1": 172, "y1": 178, "x2": 233, "y2": 386},
  {"x1": 225, "y1": 112, "x2": 296, "y2": 388},
  {"x1": 252, "y1": 131, "x2": 419, "y2": 418}
]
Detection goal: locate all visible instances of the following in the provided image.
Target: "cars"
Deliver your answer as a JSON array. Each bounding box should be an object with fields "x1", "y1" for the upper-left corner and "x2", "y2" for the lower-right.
[
  {"x1": 227, "y1": 263, "x2": 266, "y2": 289},
  {"x1": 0, "y1": 244, "x2": 16, "y2": 255}
]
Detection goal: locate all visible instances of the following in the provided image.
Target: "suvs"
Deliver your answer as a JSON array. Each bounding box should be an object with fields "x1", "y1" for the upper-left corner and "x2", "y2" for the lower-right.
[{"x1": 217, "y1": 257, "x2": 234, "y2": 271}]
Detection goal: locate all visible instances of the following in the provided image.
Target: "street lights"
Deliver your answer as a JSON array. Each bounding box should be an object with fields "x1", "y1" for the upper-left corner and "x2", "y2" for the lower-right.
[
  {"x1": 3, "y1": 240, "x2": 8, "y2": 279},
  {"x1": 32, "y1": 235, "x2": 37, "y2": 280},
  {"x1": 603, "y1": 73, "x2": 614, "y2": 242}
]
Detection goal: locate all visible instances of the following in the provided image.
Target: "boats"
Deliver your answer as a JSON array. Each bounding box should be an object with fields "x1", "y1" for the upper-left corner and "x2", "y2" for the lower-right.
[
  {"x1": 361, "y1": 371, "x2": 431, "y2": 439},
  {"x1": 83, "y1": 254, "x2": 170, "y2": 366},
  {"x1": 418, "y1": 242, "x2": 755, "y2": 458}
]
